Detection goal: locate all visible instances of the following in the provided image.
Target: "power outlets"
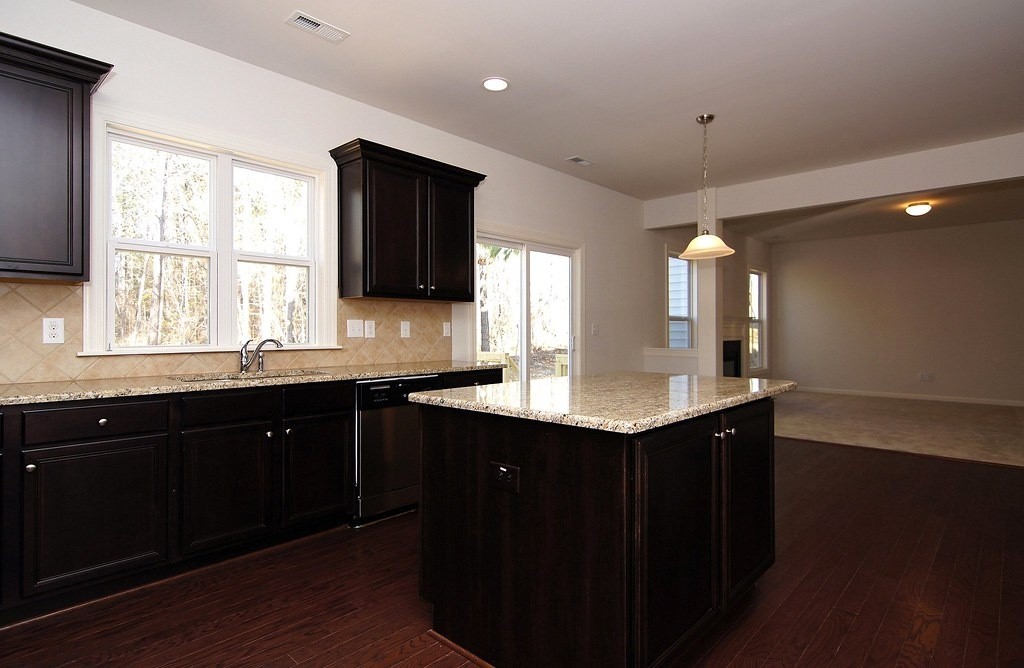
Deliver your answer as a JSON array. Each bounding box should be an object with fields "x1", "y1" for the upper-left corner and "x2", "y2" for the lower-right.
[
  {"x1": 443, "y1": 322, "x2": 451, "y2": 336},
  {"x1": 42, "y1": 318, "x2": 64, "y2": 343},
  {"x1": 364, "y1": 321, "x2": 376, "y2": 338},
  {"x1": 347, "y1": 320, "x2": 363, "y2": 337},
  {"x1": 488, "y1": 462, "x2": 520, "y2": 493},
  {"x1": 401, "y1": 321, "x2": 410, "y2": 337}
]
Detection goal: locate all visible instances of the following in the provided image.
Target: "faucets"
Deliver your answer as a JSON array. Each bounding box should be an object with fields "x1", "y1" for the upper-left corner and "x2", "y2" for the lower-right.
[{"x1": 240, "y1": 338, "x2": 284, "y2": 371}]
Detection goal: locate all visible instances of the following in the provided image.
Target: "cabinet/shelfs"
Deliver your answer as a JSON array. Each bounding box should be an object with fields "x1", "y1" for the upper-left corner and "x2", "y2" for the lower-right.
[
  {"x1": 176, "y1": 380, "x2": 356, "y2": 576},
  {"x1": 328, "y1": 138, "x2": 487, "y2": 302},
  {"x1": 0, "y1": 393, "x2": 177, "y2": 628},
  {"x1": 0, "y1": 30, "x2": 113, "y2": 286},
  {"x1": 418, "y1": 396, "x2": 777, "y2": 668},
  {"x1": 440, "y1": 368, "x2": 503, "y2": 389}
]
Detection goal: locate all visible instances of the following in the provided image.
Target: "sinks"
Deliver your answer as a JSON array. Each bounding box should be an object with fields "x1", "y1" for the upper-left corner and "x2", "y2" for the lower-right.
[{"x1": 170, "y1": 369, "x2": 331, "y2": 385}]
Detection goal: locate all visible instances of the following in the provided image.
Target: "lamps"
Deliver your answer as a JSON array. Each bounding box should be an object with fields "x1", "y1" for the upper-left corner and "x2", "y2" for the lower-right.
[
  {"x1": 905, "y1": 202, "x2": 932, "y2": 216},
  {"x1": 678, "y1": 113, "x2": 735, "y2": 259}
]
